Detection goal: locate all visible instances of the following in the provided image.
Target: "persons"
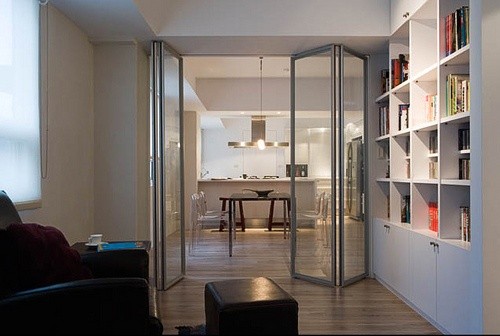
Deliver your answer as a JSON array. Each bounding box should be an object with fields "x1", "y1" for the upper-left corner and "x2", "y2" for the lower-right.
[{"x1": 0, "y1": 224, "x2": 163, "y2": 336}]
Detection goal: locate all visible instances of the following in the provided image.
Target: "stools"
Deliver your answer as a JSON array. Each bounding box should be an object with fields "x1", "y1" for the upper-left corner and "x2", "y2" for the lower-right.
[
  {"x1": 202, "y1": 275, "x2": 300, "y2": 336},
  {"x1": 266, "y1": 198, "x2": 292, "y2": 232},
  {"x1": 219, "y1": 193, "x2": 247, "y2": 232}
]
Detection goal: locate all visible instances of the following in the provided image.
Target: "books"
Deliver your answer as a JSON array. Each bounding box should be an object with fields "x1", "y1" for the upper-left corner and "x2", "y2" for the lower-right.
[
  {"x1": 376, "y1": 139, "x2": 390, "y2": 178},
  {"x1": 387, "y1": 195, "x2": 390, "y2": 219},
  {"x1": 406, "y1": 135, "x2": 410, "y2": 178},
  {"x1": 425, "y1": 95, "x2": 437, "y2": 123},
  {"x1": 458, "y1": 121, "x2": 470, "y2": 180},
  {"x1": 399, "y1": 103, "x2": 410, "y2": 131},
  {"x1": 428, "y1": 130, "x2": 438, "y2": 179},
  {"x1": 381, "y1": 69, "x2": 389, "y2": 96},
  {"x1": 460, "y1": 206, "x2": 470, "y2": 242},
  {"x1": 391, "y1": 54, "x2": 408, "y2": 89},
  {"x1": 429, "y1": 201, "x2": 438, "y2": 232},
  {"x1": 445, "y1": 6, "x2": 470, "y2": 57},
  {"x1": 401, "y1": 195, "x2": 410, "y2": 223},
  {"x1": 379, "y1": 106, "x2": 389, "y2": 136},
  {"x1": 445, "y1": 73, "x2": 470, "y2": 116}
]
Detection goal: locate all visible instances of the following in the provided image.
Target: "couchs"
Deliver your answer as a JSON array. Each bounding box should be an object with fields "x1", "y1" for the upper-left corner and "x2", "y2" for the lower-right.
[{"x1": 0, "y1": 188, "x2": 164, "y2": 336}]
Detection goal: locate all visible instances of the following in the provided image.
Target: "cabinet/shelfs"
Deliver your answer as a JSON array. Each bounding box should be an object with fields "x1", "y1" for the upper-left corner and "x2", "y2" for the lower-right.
[{"x1": 367, "y1": 0, "x2": 500, "y2": 336}]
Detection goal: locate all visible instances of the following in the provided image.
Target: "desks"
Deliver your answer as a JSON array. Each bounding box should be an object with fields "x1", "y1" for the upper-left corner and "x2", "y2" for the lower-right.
[{"x1": 228, "y1": 191, "x2": 297, "y2": 257}]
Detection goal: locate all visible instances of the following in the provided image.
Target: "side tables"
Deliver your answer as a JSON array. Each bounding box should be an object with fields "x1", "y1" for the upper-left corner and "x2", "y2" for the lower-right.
[{"x1": 70, "y1": 240, "x2": 153, "y2": 255}]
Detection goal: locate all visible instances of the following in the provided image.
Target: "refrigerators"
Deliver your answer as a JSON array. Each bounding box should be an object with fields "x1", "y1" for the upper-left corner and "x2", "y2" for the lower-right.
[{"x1": 346, "y1": 140, "x2": 365, "y2": 220}]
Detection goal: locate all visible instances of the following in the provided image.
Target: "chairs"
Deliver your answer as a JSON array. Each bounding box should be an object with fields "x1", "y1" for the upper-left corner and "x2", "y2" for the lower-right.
[
  {"x1": 187, "y1": 190, "x2": 235, "y2": 257},
  {"x1": 287, "y1": 191, "x2": 331, "y2": 249}
]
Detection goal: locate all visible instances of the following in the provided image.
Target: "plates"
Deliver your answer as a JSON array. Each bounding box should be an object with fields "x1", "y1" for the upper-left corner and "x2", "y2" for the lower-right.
[{"x1": 85, "y1": 242, "x2": 109, "y2": 246}]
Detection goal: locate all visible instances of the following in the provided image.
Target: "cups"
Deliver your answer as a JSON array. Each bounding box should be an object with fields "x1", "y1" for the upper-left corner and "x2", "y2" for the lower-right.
[
  {"x1": 243, "y1": 174, "x2": 246, "y2": 179},
  {"x1": 88, "y1": 234, "x2": 102, "y2": 244}
]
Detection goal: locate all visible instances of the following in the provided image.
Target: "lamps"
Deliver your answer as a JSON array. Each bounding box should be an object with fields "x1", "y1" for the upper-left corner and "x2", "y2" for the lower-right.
[{"x1": 256, "y1": 56, "x2": 267, "y2": 151}]
